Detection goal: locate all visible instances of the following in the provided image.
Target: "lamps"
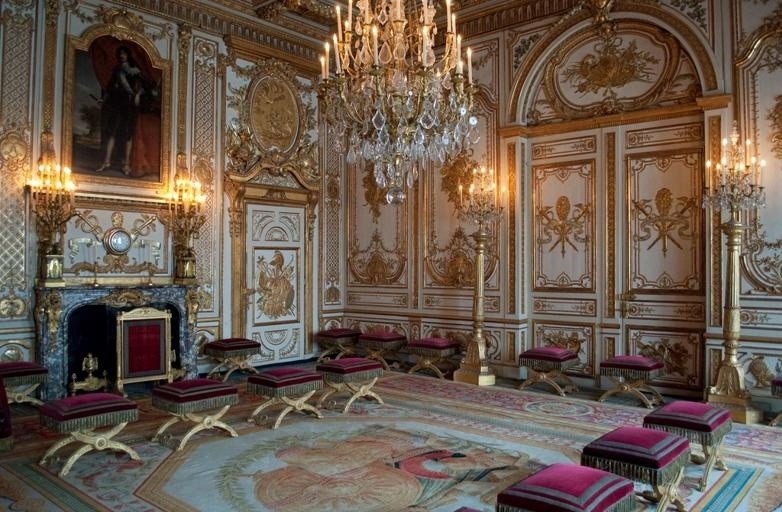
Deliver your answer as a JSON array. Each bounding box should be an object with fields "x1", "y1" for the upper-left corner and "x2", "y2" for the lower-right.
[{"x1": 314, "y1": 1, "x2": 480, "y2": 208}]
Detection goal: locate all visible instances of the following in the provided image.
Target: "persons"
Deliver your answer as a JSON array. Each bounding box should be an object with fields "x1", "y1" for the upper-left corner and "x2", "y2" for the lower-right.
[{"x1": 96, "y1": 46, "x2": 145, "y2": 176}]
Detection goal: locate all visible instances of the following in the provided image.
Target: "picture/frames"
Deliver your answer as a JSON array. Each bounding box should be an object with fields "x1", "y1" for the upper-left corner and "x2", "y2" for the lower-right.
[{"x1": 59, "y1": 10, "x2": 172, "y2": 193}]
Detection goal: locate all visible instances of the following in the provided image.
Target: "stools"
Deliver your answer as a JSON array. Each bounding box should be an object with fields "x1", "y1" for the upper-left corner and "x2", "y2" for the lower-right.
[
  {"x1": 360, "y1": 330, "x2": 407, "y2": 372},
  {"x1": 518, "y1": 347, "x2": 580, "y2": 397},
  {"x1": 38, "y1": 392, "x2": 140, "y2": 477},
  {"x1": 489, "y1": 401, "x2": 735, "y2": 511},
  {"x1": 247, "y1": 367, "x2": 322, "y2": 430},
  {"x1": 315, "y1": 357, "x2": 385, "y2": 414},
  {"x1": 314, "y1": 328, "x2": 363, "y2": 363},
  {"x1": 599, "y1": 356, "x2": 665, "y2": 409},
  {"x1": 203, "y1": 337, "x2": 261, "y2": 383},
  {"x1": 1, "y1": 361, "x2": 49, "y2": 405},
  {"x1": 408, "y1": 337, "x2": 461, "y2": 379},
  {"x1": 150, "y1": 380, "x2": 239, "y2": 452}
]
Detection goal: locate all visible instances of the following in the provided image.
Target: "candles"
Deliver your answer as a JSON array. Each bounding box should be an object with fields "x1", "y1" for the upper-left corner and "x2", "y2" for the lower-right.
[
  {"x1": 458, "y1": 167, "x2": 499, "y2": 209},
  {"x1": 34, "y1": 164, "x2": 75, "y2": 207},
  {"x1": 715, "y1": 137, "x2": 765, "y2": 186},
  {"x1": 166, "y1": 180, "x2": 206, "y2": 217}
]
